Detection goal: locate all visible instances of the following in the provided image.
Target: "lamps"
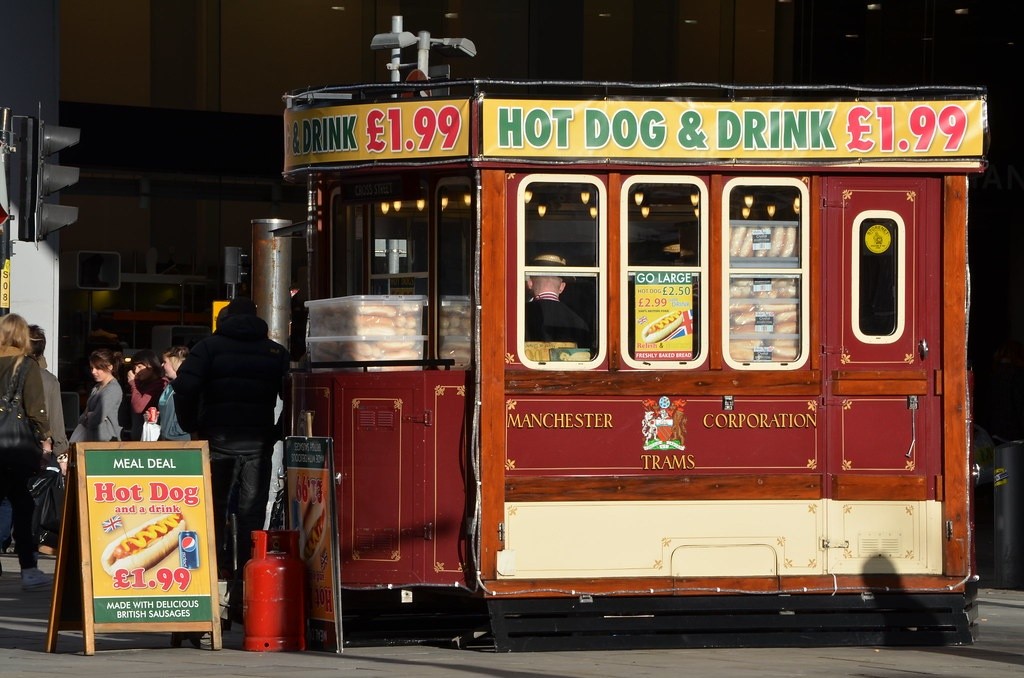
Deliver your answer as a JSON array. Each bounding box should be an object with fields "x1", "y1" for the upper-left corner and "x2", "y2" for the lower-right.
[{"x1": 369, "y1": 13, "x2": 478, "y2": 82}]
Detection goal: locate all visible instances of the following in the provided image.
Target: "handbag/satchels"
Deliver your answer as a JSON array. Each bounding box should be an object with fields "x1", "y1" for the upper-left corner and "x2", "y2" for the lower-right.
[
  {"x1": 69, "y1": 413, "x2": 112, "y2": 443},
  {"x1": 40, "y1": 467, "x2": 64, "y2": 534},
  {"x1": 0, "y1": 354, "x2": 43, "y2": 465}
]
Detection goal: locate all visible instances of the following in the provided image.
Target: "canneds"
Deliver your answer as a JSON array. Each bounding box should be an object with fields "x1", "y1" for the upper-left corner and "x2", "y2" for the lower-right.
[
  {"x1": 179, "y1": 532, "x2": 199, "y2": 570},
  {"x1": 148, "y1": 407, "x2": 158, "y2": 423}
]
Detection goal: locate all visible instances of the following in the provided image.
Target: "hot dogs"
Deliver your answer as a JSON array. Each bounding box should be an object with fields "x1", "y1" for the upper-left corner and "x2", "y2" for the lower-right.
[
  {"x1": 300, "y1": 496, "x2": 325, "y2": 560},
  {"x1": 642, "y1": 311, "x2": 683, "y2": 343},
  {"x1": 102, "y1": 512, "x2": 187, "y2": 578}
]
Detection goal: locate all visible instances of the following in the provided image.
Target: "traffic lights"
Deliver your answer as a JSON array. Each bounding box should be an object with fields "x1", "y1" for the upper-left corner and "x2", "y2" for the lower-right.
[{"x1": 30, "y1": 99, "x2": 83, "y2": 242}]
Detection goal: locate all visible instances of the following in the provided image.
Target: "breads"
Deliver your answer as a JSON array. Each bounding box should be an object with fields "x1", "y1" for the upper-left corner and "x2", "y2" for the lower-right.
[
  {"x1": 312, "y1": 302, "x2": 418, "y2": 370},
  {"x1": 439, "y1": 304, "x2": 471, "y2": 362},
  {"x1": 729, "y1": 226, "x2": 797, "y2": 257},
  {"x1": 729, "y1": 278, "x2": 797, "y2": 361}
]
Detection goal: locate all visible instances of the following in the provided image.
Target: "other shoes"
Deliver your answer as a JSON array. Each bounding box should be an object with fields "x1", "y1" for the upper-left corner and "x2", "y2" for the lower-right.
[{"x1": 21, "y1": 568, "x2": 55, "y2": 592}]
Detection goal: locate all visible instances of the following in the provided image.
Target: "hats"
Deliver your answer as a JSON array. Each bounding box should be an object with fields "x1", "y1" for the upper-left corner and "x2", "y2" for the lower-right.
[
  {"x1": 219, "y1": 297, "x2": 257, "y2": 321},
  {"x1": 523, "y1": 244, "x2": 576, "y2": 283}
]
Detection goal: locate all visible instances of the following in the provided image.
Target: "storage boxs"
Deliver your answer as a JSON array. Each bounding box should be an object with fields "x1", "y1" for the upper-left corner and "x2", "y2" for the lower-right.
[
  {"x1": 729, "y1": 332, "x2": 800, "y2": 362},
  {"x1": 438, "y1": 336, "x2": 471, "y2": 371},
  {"x1": 728, "y1": 220, "x2": 799, "y2": 269},
  {"x1": 729, "y1": 274, "x2": 800, "y2": 300},
  {"x1": 437, "y1": 296, "x2": 472, "y2": 336},
  {"x1": 304, "y1": 295, "x2": 429, "y2": 336},
  {"x1": 728, "y1": 298, "x2": 799, "y2": 334},
  {"x1": 306, "y1": 335, "x2": 428, "y2": 371}
]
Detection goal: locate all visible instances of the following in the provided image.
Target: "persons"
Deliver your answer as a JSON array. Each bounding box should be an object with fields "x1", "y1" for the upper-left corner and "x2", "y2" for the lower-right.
[
  {"x1": 525, "y1": 250, "x2": 589, "y2": 348},
  {"x1": 143, "y1": 346, "x2": 191, "y2": 441},
  {"x1": 0, "y1": 325, "x2": 69, "y2": 553},
  {"x1": 69, "y1": 349, "x2": 123, "y2": 442},
  {"x1": 0, "y1": 313, "x2": 55, "y2": 589},
  {"x1": 126, "y1": 350, "x2": 165, "y2": 441},
  {"x1": 173, "y1": 296, "x2": 292, "y2": 624}
]
